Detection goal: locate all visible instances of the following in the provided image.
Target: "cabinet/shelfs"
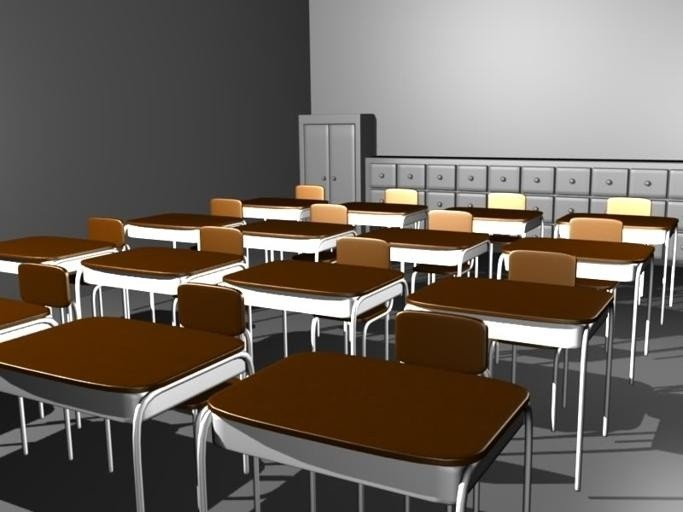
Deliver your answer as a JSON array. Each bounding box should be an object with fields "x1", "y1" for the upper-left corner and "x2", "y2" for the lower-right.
[{"x1": 297, "y1": 113, "x2": 683, "y2": 260}]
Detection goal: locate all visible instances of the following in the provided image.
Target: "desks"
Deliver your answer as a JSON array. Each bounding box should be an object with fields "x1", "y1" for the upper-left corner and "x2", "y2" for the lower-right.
[{"x1": 0, "y1": 197, "x2": 680, "y2": 512}]
